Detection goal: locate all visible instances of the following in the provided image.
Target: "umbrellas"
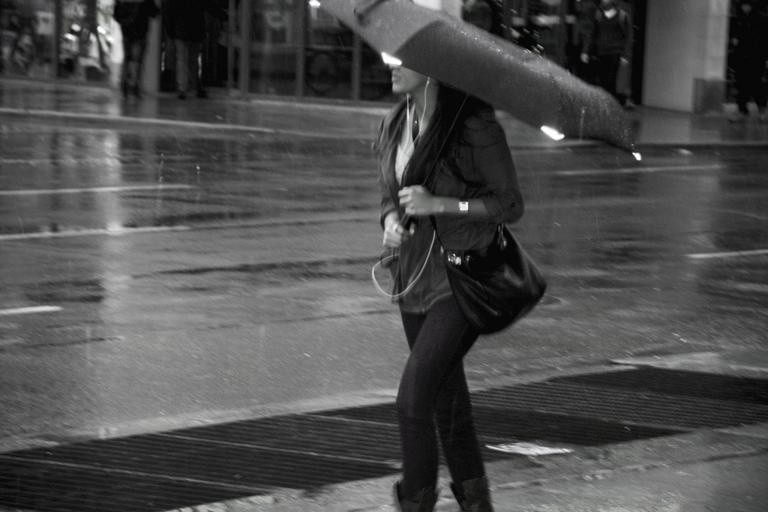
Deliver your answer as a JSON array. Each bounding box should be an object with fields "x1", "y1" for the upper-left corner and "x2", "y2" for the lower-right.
[{"x1": 319, "y1": 0, "x2": 637, "y2": 225}]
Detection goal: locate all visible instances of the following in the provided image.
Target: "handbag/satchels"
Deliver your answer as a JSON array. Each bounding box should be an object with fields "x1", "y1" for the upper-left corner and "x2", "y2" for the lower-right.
[{"x1": 442, "y1": 221, "x2": 547, "y2": 334}]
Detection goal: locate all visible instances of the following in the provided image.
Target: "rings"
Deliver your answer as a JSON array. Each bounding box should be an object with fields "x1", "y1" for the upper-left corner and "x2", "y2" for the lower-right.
[{"x1": 394, "y1": 224, "x2": 399, "y2": 232}]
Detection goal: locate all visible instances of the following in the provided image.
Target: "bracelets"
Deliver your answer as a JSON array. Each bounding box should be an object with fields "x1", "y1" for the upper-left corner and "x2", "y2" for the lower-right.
[
  {"x1": 457, "y1": 197, "x2": 470, "y2": 216},
  {"x1": 436, "y1": 198, "x2": 446, "y2": 214}
]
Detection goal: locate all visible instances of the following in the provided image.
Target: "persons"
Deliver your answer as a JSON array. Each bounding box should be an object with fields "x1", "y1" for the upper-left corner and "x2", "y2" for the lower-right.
[
  {"x1": 163, "y1": 0, "x2": 228, "y2": 100},
  {"x1": 372, "y1": 63, "x2": 524, "y2": 512},
  {"x1": 728, "y1": 1, "x2": 768, "y2": 124},
  {"x1": 112, "y1": 0, "x2": 160, "y2": 98},
  {"x1": 562, "y1": 0, "x2": 634, "y2": 99}
]
